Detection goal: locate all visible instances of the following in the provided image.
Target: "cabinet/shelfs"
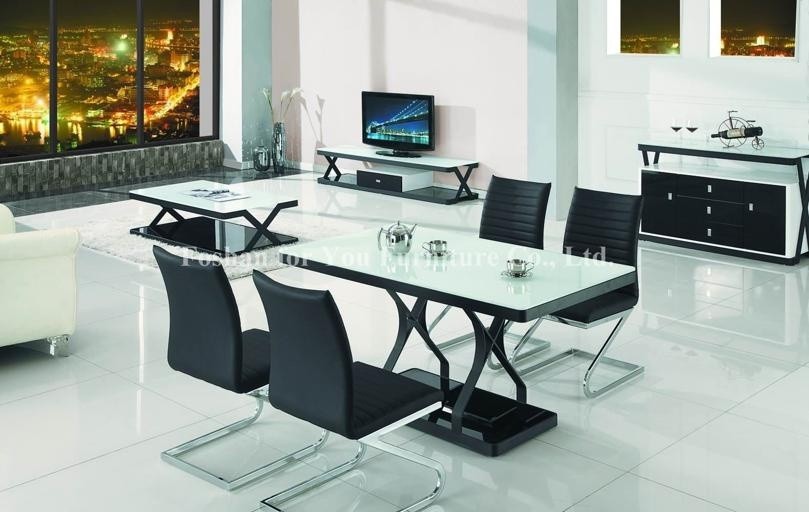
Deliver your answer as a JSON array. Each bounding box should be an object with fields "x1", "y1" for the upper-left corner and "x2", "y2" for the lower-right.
[{"x1": 640, "y1": 172, "x2": 787, "y2": 256}]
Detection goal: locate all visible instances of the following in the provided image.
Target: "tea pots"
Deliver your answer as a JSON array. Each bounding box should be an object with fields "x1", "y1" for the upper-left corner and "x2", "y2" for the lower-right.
[{"x1": 377, "y1": 220, "x2": 417, "y2": 256}]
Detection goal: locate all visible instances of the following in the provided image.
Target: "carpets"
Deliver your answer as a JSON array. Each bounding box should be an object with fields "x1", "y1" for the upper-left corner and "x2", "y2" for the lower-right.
[{"x1": 80, "y1": 209, "x2": 317, "y2": 280}]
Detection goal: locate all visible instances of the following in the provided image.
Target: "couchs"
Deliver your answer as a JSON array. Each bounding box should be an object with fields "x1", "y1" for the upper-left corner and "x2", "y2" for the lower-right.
[{"x1": 0, "y1": 204, "x2": 78, "y2": 359}]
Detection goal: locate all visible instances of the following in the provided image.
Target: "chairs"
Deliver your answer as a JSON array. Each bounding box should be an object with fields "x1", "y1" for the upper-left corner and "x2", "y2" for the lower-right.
[
  {"x1": 152, "y1": 244, "x2": 328, "y2": 493},
  {"x1": 507, "y1": 186, "x2": 645, "y2": 401},
  {"x1": 247, "y1": 270, "x2": 449, "y2": 512},
  {"x1": 426, "y1": 173, "x2": 551, "y2": 371}
]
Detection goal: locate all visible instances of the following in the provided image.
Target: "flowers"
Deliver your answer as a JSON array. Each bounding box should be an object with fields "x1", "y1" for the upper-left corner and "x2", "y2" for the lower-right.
[{"x1": 262, "y1": 86, "x2": 305, "y2": 121}]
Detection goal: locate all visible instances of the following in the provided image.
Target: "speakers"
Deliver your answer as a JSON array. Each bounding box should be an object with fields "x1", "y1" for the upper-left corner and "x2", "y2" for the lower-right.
[{"x1": 357, "y1": 168, "x2": 434, "y2": 193}]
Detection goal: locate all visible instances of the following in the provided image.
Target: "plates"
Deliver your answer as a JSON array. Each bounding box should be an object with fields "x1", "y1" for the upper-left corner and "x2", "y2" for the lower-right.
[
  {"x1": 423, "y1": 252, "x2": 452, "y2": 260},
  {"x1": 501, "y1": 270, "x2": 532, "y2": 281}
]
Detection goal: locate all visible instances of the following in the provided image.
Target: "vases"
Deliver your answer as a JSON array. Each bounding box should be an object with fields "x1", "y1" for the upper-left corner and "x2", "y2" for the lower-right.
[{"x1": 272, "y1": 121, "x2": 287, "y2": 174}]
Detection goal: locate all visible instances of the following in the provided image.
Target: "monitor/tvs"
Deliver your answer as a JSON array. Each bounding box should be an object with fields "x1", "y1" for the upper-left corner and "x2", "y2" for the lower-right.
[{"x1": 361, "y1": 92, "x2": 435, "y2": 158}]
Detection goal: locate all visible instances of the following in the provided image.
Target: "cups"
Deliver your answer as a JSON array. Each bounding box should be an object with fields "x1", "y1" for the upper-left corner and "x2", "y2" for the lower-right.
[
  {"x1": 423, "y1": 241, "x2": 447, "y2": 256},
  {"x1": 507, "y1": 259, "x2": 534, "y2": 275}
]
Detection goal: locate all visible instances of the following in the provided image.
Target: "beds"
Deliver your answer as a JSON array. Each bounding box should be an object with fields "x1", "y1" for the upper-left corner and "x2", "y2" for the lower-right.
[{"x1": 315, "y1": 143, "x2": 481, "y2": 208}]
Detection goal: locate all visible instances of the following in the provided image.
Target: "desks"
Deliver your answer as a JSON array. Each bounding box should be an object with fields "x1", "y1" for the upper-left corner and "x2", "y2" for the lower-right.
[{"x1": 636, "y1": 136, "x2": 809, "y2": 266}]
